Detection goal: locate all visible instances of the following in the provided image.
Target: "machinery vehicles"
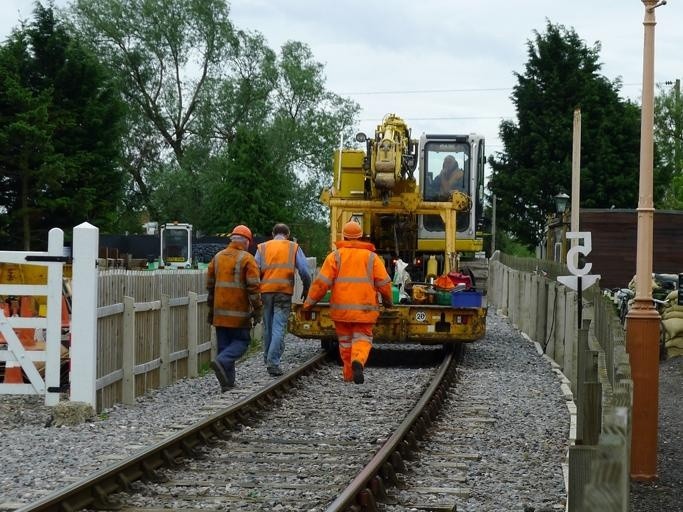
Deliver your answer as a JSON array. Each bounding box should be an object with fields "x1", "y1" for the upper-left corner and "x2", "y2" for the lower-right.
[
  {"x1": 280, "y1": 112, "x2": 504, "y2": 358},
  {"x1": 155, "y1": 219, "x2": 197, "y2": 272}
]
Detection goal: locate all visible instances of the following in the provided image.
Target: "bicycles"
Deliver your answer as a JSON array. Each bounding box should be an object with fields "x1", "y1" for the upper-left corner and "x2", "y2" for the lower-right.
[
  {"x1": 611, "y1": 287, "x2": 633, "y2": 324},
  {"x1": 650, "y1": 295, "x2": 670, "y2": 358}
]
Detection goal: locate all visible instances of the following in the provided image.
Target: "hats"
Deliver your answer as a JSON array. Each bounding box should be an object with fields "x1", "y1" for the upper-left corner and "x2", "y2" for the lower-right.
[
  {"x1": 230, "y1": 225, "x2": 252, "y2": 240},
  {"x1": 342, "y1": 222, "x2": 364, "y2": 239}
]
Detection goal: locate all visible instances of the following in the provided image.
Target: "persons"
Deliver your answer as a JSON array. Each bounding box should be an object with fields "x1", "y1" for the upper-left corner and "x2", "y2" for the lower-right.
[
  {"x1": 433, "y1": 156, "x2": 464, "y2": 201},
  {"x1": 205, "y1": 224, "x2": 262, "y2": 392},
  {"x1": 304, "y1": 219, "x2": 393, "y2": 387},
  {"x1": 253, "y1": 224, "x2": 314, "y2": 376}
]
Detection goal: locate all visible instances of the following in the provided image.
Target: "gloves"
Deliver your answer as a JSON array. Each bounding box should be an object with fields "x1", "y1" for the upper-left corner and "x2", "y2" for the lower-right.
[
  {"x1": 252, "y1": 299, "x2": 266, "y2": 326},
  {"x1": 382, "y1": 300, "x2": 393, "y2": 310},
  {"x1": 300, "y1": 284, "x2": 311, "y2": 303},
  {"x1": 207, "y1": 303, "x2": 216, "y2": 326}
]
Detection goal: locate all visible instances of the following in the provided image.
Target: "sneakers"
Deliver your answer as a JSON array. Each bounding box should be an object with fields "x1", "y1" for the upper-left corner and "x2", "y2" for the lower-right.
[
  {"x1": 209, "y1": 358, "x2": 234, "y2": 387},
  {"x1": 265, "y1": 367, "x2": 284, "y2": 377},
  {"x1": 352, "y1": 360, "x2": 365, "y2": 386}
]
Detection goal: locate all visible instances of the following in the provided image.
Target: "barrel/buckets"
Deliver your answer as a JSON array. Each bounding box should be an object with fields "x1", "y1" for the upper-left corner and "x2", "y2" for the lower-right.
[
  {"x1": 390, "y1": 280, "x2": 399, "y2": 303},
  {"x1": 452, "y1": 288, "x2": 482, "y2": 307}
]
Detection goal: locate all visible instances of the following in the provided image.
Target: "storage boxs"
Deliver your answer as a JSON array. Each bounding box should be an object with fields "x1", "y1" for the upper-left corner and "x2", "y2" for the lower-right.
[{"x1": 450, "y1": 291, "x2": 482, "y2": 307}]
